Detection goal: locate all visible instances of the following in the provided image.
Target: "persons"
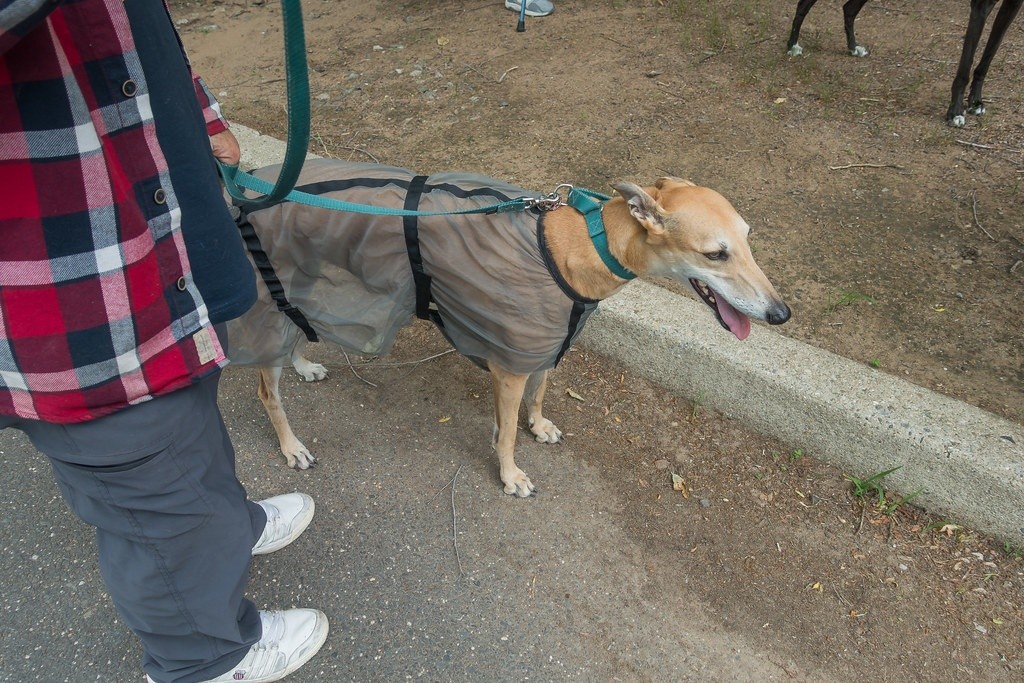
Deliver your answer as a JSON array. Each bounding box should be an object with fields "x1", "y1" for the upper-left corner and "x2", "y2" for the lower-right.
[{"x1": 0, "y1": 0, "x2": 329, "y2": 683}]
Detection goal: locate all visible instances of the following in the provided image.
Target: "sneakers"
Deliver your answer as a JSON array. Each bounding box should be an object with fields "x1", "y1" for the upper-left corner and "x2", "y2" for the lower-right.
[
  {"x1": 504, "y1": 0, "x2": 556, "y2": 17},
  {"x1": 145, "y1": 608, "x2": 330, "y2": 683},
  {"x1": 248, "y1": 492, "x2": 316, "y2": 558}
]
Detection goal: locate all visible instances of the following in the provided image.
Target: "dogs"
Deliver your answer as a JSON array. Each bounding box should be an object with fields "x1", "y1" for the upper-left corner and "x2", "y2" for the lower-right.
[
  {"x1": 220, "y1": 160, "x2": 791, "y2": 499},
  {"x1": 786, "y1": 0, "x2": 1024, "y2": 130}
]
